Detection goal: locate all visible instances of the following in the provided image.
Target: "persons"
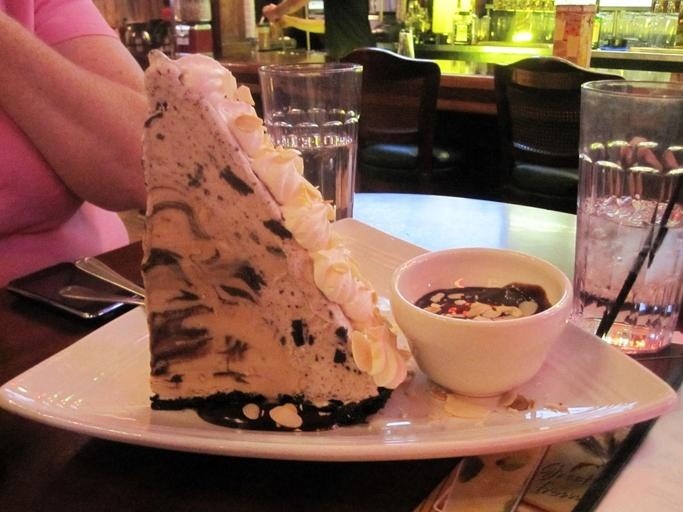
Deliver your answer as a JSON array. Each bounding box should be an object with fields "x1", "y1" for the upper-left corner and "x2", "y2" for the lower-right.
[
  {"x1": 261, "y1": 0, "x2": 377, "y2": 60},
  {"x1": 0, "y1": 0, "x2": 147, "y2": 289},
  {"x1": 157, "y1": 0, "x2": 175, "y2": 24}
]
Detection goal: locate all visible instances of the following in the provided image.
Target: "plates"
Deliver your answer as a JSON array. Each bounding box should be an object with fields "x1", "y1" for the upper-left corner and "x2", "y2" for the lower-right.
[{"x1": 0, "y1": 215, "x2": 681, "y2": 462}]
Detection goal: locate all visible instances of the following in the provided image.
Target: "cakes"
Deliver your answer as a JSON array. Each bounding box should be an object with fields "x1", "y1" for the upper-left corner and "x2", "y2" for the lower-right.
[{"x1": 141, "y1": 46, "x2": 411, "y2": 431}]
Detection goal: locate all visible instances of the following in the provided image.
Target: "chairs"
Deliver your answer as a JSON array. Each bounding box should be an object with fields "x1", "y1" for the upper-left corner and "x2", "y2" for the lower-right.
[
  {"x1": 339, "y1": 47, "x2": 458, "y2": 192},
  {"x1": 494, "y1": 57, "x2": 626, "y2": 214}
]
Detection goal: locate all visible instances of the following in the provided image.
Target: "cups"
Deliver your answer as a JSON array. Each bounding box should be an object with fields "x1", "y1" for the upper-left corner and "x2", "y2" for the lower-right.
[
  {"x1": 258, "y1": 61, "x2": 366, "y2": 227},
  {"x1": 601, "y1": 8, "x2": 680, "y2": 47},
  {"x1": 572, "y1": 78, "x2": 682, "y2": 357}
]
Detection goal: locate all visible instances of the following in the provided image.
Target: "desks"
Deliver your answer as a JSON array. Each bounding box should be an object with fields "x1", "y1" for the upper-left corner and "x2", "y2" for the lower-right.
[{"x1": 217, "y1": 60, "x2": 683, "y2": 124}]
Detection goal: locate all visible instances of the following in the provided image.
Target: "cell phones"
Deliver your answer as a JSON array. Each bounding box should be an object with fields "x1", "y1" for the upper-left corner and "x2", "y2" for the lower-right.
[{"x1": 9, "y1": 260, "x2": 139, "y2": 328}]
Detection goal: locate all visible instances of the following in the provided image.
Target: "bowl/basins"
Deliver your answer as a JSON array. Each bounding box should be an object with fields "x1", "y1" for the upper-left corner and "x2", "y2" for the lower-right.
[{"x1": 388, "y1": 246, "x2": 575, "y2": 399}]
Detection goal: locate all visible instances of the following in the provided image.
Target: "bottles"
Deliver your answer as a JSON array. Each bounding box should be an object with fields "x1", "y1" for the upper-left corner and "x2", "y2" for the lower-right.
[
  {"x1": 451, "y1": 3, "x2": 498, "y2": 47},
  {"x1": 589, "y1": 1, "x2": 603, "y2": 51},
  {"x1": 257, "y1": 14, "x2": 273, "y2": 51},
  {"x1": 125, "y1": 23, "x2": 150, "y2": 63},
  {"x1": 153, "y1": 8, "x2": 178, "y2": 61}
]
